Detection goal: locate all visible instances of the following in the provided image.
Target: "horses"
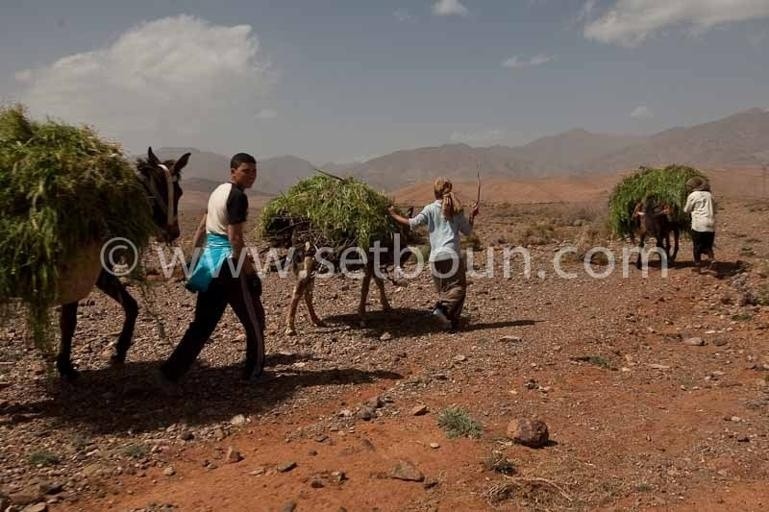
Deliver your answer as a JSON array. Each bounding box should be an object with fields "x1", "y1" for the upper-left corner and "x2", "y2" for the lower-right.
[
  {"x1": 637, "y1": 192, "x2": 683, "y2": 268},
  {"x1": 0, "y1": 146, "x2": 192, "y2": 383},
  {"x1": 284, "y1": 205, "x2": 415, "y2": 336}
]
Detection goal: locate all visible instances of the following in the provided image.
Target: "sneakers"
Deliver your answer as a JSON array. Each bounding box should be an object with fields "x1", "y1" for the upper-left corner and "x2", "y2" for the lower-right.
[
  {"x1": 155, "y1": 366, "x2": 183, "y2": 396},
  {"x1": 693, "y1": 258, "x2": 718, "y2": 271},
  {"x1": 431, "y1": 305, "x2": 454, "y2": 330},
  {"x1": 240, "y1": 373, "x2": 278, "y2": 385}
]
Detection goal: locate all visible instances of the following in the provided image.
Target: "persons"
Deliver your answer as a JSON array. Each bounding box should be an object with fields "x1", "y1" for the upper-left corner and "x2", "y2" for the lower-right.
[
  {"x1": 386, "y1": 173, "x2": 482, "y2": 332},
  {"x1": 155, "y1": 151, "x2": 283, "y2": 398},
  {"x1": 683, "y1": 175, "x2": 717, "y2": 266}
]
